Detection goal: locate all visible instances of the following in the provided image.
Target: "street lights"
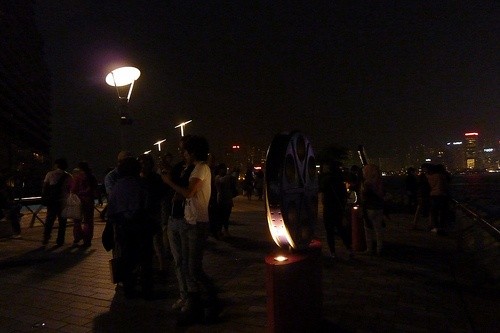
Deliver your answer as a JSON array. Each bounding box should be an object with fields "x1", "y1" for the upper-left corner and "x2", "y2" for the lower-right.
[{"x1": 104, "y1": 63, "x2": 142, "y2": 151}]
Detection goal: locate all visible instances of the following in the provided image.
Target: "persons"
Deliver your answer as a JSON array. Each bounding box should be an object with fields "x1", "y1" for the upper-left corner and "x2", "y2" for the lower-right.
[
  {"x1": 6, "y1": 134, "x2": 264, "y2": 318},
  {"x1": 318, "y1": 157, "x2": 455, "y2": 263}
]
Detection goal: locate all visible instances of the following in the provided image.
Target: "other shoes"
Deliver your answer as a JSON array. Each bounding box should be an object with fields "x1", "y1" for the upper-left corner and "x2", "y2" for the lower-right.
[
  {"x1": 171, "y1": 298, "x2": 185, "y2": 310},
  {"x1": 81, "y1": 244, "x2": 92, "y2": 249},
  {"x1": 56, "y1": 241, "x2": 65, "y2": 247},
  {"x1": 41, "y1": 240, "x2": 49, "y2": 246},
  {"x1": 72, "y1": 242, "x2": 81, "y2": 247},
  {"x1": 181, "y1": 296, "x2": 196, "y2": 312},
  {"x1": 12, "y1": 235, "x2": 23, "y2": 239}
]
